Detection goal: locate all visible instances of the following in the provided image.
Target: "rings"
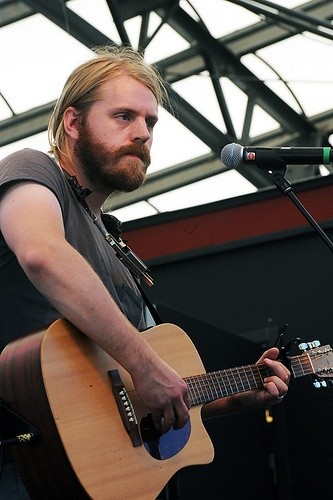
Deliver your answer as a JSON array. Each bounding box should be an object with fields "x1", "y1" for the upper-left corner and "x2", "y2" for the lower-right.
[{"x1": 277, "y1": 395, "x2": 286, "y2": 399}]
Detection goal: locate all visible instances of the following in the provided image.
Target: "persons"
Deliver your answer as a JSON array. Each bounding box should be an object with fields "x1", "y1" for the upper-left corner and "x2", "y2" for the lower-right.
[{"x1": 0, "y1": 46, "x2": 295, "y2": 500}]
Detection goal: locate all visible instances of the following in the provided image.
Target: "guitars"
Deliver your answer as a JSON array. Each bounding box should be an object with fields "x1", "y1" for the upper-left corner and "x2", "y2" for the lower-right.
[{"x1": 0, "y1": 317, "x2": 333, "y2": 500}]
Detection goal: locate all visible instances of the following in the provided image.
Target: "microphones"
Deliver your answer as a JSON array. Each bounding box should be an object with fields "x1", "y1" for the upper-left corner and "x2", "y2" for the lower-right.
[{"x1": 219, "y1": 140, "x2": 333, "y2": 168}]
